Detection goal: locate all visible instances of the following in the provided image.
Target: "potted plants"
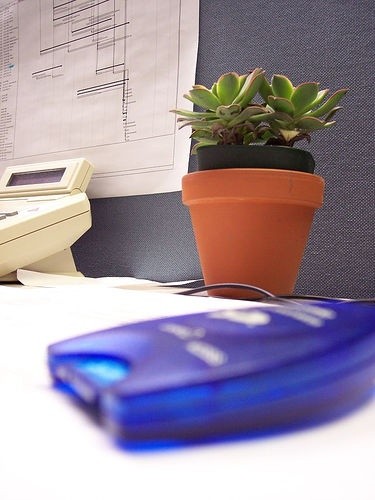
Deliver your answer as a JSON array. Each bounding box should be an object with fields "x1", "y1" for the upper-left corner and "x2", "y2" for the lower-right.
[{"x1": 168, "y1": 67, "x2": 349, "y2": 299}]
